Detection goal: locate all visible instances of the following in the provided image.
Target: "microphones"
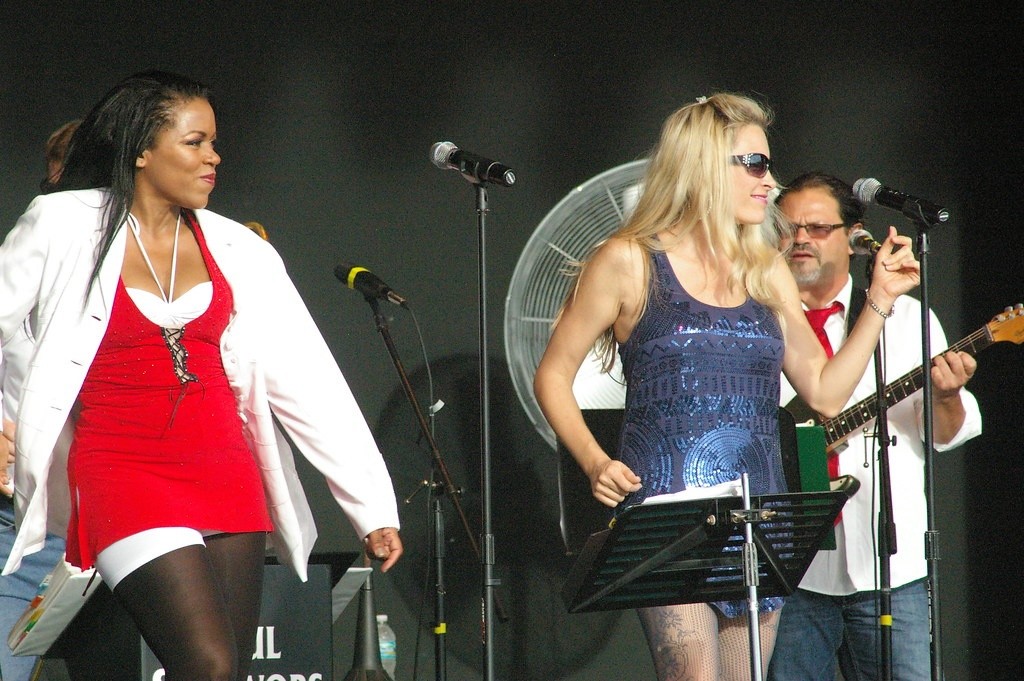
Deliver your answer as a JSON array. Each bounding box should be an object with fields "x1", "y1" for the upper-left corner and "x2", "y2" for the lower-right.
[
  {"x1": 851, "y1": 178, "x2": 948, "y2": 227},
  {"x1": 429, "y1": 139, "x2": 516, "y2": 188},
  {"x1": 333, "y1": 264, "x2": 409, "y2": 309}
]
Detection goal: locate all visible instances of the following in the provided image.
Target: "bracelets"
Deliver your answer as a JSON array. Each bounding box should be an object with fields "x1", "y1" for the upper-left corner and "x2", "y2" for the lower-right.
[{"x1": 864, "y1": 286, "x2": 896, "y2": 319}]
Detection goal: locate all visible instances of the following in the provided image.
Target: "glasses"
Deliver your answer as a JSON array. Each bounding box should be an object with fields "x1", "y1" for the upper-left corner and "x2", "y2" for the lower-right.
[
  {"x1": 728, "y1": 152, "x2": 773, "y2": 178},
  {"x1": 778, "y1": 223, "x2": 849, "y2": 240}
]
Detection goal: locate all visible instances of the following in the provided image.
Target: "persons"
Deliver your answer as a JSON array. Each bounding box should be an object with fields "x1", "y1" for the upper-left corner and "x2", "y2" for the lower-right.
[
  {"x1": 765, "y1": 170, "x2": 982, "y2": 681},
  {"x1": 533, "y1": 92, "x2": 920, "y2": 680},
  {"x1": 1, "y1": 67, "x2": 404, "y2": 681}
]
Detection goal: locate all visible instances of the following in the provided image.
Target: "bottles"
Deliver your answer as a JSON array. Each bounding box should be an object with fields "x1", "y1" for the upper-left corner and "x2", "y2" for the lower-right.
[{"x1": 376, "y1": 614, "x2": 396, "y2": 680}]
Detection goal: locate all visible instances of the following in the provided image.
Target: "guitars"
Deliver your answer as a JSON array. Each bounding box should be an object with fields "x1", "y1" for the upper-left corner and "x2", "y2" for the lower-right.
[{"x1": 811, "y1": 299, "x2": 1024, "y2": 451}]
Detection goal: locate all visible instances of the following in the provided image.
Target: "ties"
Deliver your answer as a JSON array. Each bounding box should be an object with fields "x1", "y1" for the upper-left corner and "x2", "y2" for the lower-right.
[{"x1": 802, "y1": 299, "x2": 844, "y2": 530}]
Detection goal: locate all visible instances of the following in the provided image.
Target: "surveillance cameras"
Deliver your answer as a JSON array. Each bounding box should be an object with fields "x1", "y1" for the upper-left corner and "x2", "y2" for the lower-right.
[{"x1": 849, "y1": 230, "x2": 882, "y2": 252}]
